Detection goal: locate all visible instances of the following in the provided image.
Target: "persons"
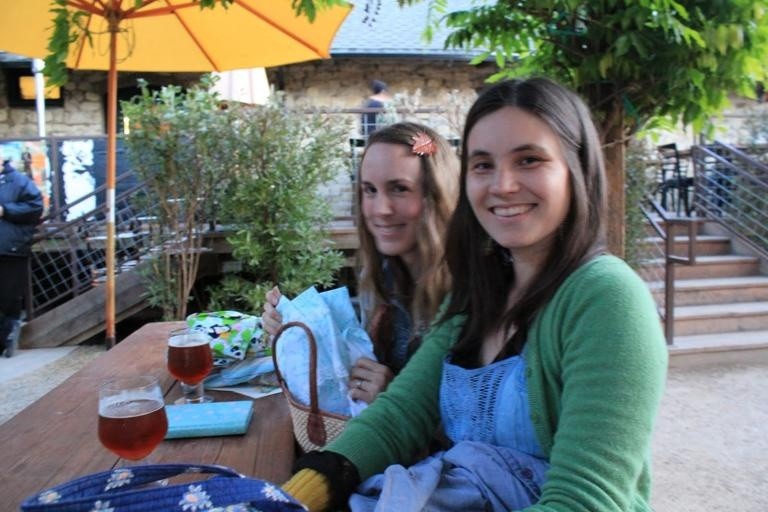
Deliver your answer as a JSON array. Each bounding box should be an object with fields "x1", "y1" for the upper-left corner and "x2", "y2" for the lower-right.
[
  {"x1": 258, "y1": 118, "x2": 462, "y2": 411},
  {"x1": 272, "y1": 72, "x2": 672, "y2": 512},
  {"x1": 0, "y1": 148, "x2": 43, "y2": 360},
  {"x1": 357, "y1": 77, "x2": 388, "y2": 136}
]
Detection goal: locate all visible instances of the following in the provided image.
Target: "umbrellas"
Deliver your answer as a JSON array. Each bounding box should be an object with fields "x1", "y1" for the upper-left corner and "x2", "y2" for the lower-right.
[{"x1": 0, "y1": 0, "x2": 353, "y2": 350}]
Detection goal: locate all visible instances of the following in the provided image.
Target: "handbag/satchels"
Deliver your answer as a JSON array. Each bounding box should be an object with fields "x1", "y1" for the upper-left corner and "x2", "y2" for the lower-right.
[{"x1": 271, "y1": 299, "x2": 415, "y2": 457}]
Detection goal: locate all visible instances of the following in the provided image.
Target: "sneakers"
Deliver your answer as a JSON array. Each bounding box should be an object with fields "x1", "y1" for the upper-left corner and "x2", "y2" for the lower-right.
[{"x1": 5, "y1": 320, "x2": 23, "y2": 358}]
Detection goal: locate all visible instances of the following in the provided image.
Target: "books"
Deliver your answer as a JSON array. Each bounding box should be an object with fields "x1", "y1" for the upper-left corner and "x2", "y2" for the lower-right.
[{"x1": 162, "y1": 399, "x2": 257, "y2": 440}]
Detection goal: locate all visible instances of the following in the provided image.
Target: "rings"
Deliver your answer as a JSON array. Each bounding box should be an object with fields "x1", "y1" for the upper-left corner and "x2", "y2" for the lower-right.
[{"x1": 356, "y1": 379, "x2": 362, "y2": 390}]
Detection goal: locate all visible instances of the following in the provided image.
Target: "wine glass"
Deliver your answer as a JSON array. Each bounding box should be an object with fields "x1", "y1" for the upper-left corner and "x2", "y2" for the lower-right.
[
  {"x1": 96, "y1": 375, "x2": 169, "y2": 464},
  {"x1": 168, "y1": 332, "x2": 216, "y2": 405}
]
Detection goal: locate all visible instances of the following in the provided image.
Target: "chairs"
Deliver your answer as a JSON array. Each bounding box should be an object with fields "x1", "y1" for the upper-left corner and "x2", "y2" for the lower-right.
[{"x1": 657, "y1": 143, "x2": 694, "y2": 218}]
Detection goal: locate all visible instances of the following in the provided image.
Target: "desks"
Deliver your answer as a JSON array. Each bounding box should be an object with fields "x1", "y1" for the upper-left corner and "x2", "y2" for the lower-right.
[{"x1": 664, "y1": 144, "x2": 768, "y2": 217}]
